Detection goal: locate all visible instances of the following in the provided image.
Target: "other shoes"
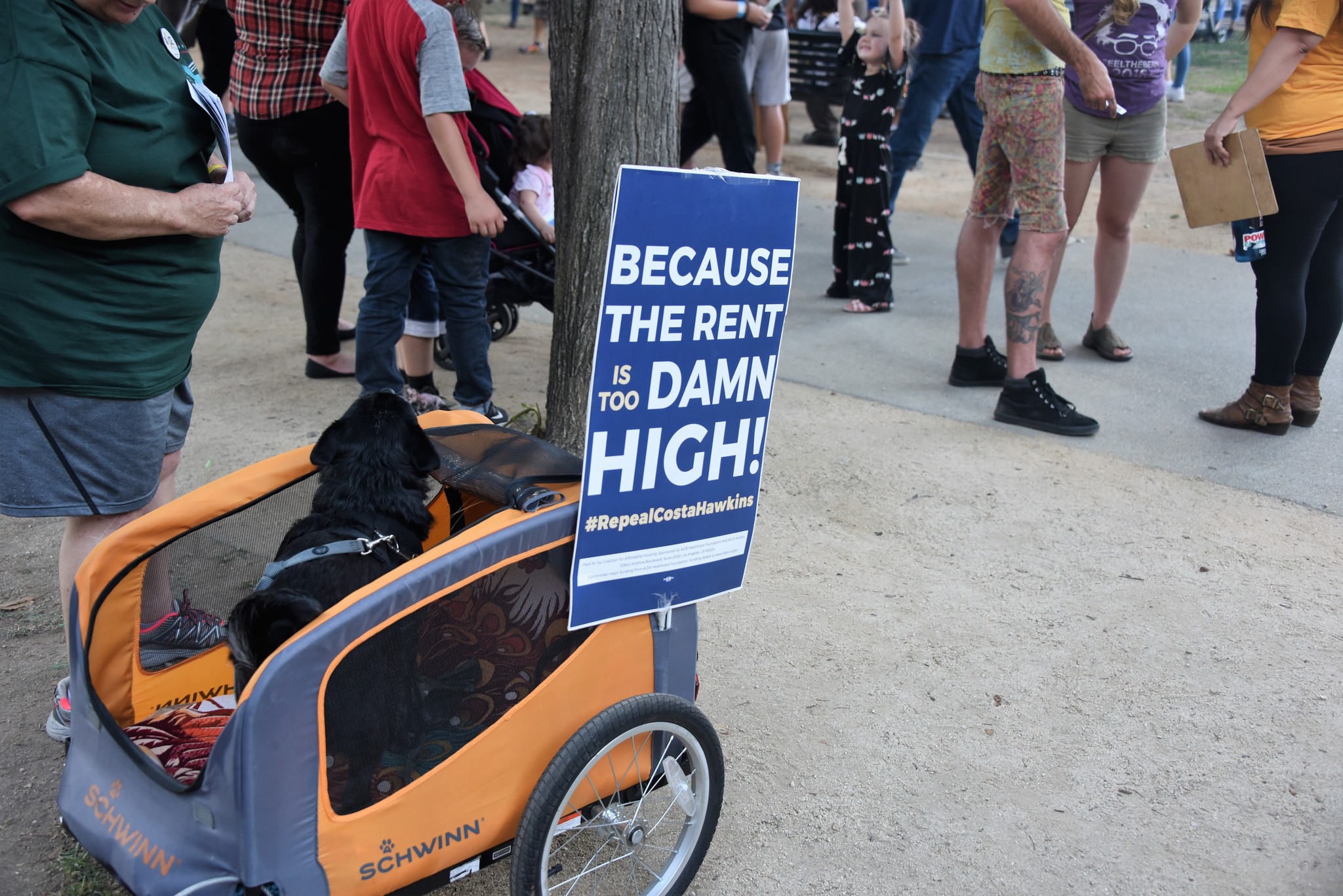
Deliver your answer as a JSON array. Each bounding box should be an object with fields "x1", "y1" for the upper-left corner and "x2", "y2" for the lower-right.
[
  {"x1": 893, "y1": 250, "x2": 910, "y2": 266},
  {"x1": 519, "y1": 43, "x2": 546, "y2": 55},
  {"x1": 1166, "y1": 85, "x2": 1185, "y2": 101},
  {"x1": 339, "y1": 326, "x2": 357, "y2": 340},
  {"x1": 803, "y1": 130, "x2": 839, "y2": 145},
  {"x1": 485, "y1": 403, "x2": 510, "y2": 427},
  {"x1": 1225, "y1": 28, "x2": 1235, "y2": 39},
  {"x1": 843, "y1": 299, "x2": 891, "y2": 313},
  {"x1": 303, "y1": 357, "x2": 356, "y2": 379},
  {"x1": 483, "y1": 47, "x2": 491, "y2": 60}
]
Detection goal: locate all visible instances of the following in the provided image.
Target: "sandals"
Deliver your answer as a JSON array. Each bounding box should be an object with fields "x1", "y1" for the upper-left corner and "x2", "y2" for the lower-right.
[
  {"x1": 1036, "y1": 323, "x2": 1066, "y2": 360},
  {"x1": 1083, "y1": 315, "x2": 1136, "y2": 361}
]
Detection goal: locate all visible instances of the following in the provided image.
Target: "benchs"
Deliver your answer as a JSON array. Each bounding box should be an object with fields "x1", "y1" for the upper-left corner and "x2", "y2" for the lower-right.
[{"x1": 788, "y1": 28, "x2": 846, "y2": 102}]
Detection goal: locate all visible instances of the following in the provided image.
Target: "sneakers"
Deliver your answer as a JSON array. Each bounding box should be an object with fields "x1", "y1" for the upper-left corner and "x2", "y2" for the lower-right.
[
  {"x1": 45, "y1": 675, "x2": 73, "y2": 739},
  {"x1": 993, "y1": 368, "x2": 1101, "y2": 435},
  {"x1": 137, "y1": 588, "x2": 229, "y2": 668},
  {"x1": 949, "y1": 334, "x2": 1009, "y2": 386}
]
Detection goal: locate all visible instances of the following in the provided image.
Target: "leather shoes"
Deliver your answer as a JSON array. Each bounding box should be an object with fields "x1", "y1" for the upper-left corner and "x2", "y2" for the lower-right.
[
  {"x1": 1290, "y1": 372, "x2": 1323, "y2": 428},
  {"x1": 1197, "y1": 376, "x2": 1294, "y2": 436}
]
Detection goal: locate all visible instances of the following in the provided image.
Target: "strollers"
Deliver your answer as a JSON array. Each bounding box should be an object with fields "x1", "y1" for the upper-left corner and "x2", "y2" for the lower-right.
[{"x1": 432, "y1": 65, "x2": 556, "y2": 372}]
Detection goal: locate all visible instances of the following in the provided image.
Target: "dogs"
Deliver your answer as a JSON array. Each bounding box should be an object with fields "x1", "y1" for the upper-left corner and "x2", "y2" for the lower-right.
[{"x1": 225, "y1": 386, "x2": 446, "y2": 819}]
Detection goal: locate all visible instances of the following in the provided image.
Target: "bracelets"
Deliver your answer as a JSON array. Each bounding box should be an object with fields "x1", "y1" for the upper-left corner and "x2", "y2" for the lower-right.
[{"x1": 208, "y1": 163, "x2": 225, "y2": 174}]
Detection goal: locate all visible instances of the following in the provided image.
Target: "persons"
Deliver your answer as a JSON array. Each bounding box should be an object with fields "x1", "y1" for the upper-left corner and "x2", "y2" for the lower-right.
[
  {"x1": 0, "y1": 0, "x2": 256, "y2": 741},
  {"x1": 519, "y1": 1, "x2": 553, "y2": 56},
  {"x1": 226, "y1": 1, "x2": 371, "y2": 380},
  {"x1": 392, "y1": 3, "x2": 486, "y2": 405},
  {"x1": 1036, "y1": 0, "x2": 1200, "y2": 362},
  {"x1": 509, "y1": 111, "x2": 562, "y2": 242},
  {"x1": 1200, "y1": 0, "x2": 1342, "y2": 436},
  {"x1": 671, "y1": 49, "x2": 698, "y2": 171},
  {"x1": 678, "y1": 0, "x2": 773, "y2": 176},
  {"x1": 883, "y1": 1, "x2": 1075, "y2": 266},
  {"x1": 742, "y1": 0, "x2": 793, "y2": 180},
  {"x1": 1158, "y1": 0, "x2": 1191, "y2": 103},
  {"x1": 948, "y1": 0, "x2": 1117, "y2": 437},
  {"x1": 320, "y1": 0, "x2": 511, "y2": 425},
  {"x1": 825, "y1": 0, "x2": 917, "y2": 312},
  {"x1": 506, "y1": 1, "x2": 521, "y2": 28},
  {"x1": 1215, "y1": 0, "x2": 1243, "y2": 37},
  {"x1": 786, "y1": 0, "x2": 842, "y2": 146},
  {"x1": 151, "y1": 0, "x2": 245, "y2": 142}
]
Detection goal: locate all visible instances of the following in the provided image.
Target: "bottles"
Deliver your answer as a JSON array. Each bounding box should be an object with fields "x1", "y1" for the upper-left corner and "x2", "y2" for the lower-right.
[{"x1": 1231, "y1": 218, "x2": 1267, "y2": 262}]
